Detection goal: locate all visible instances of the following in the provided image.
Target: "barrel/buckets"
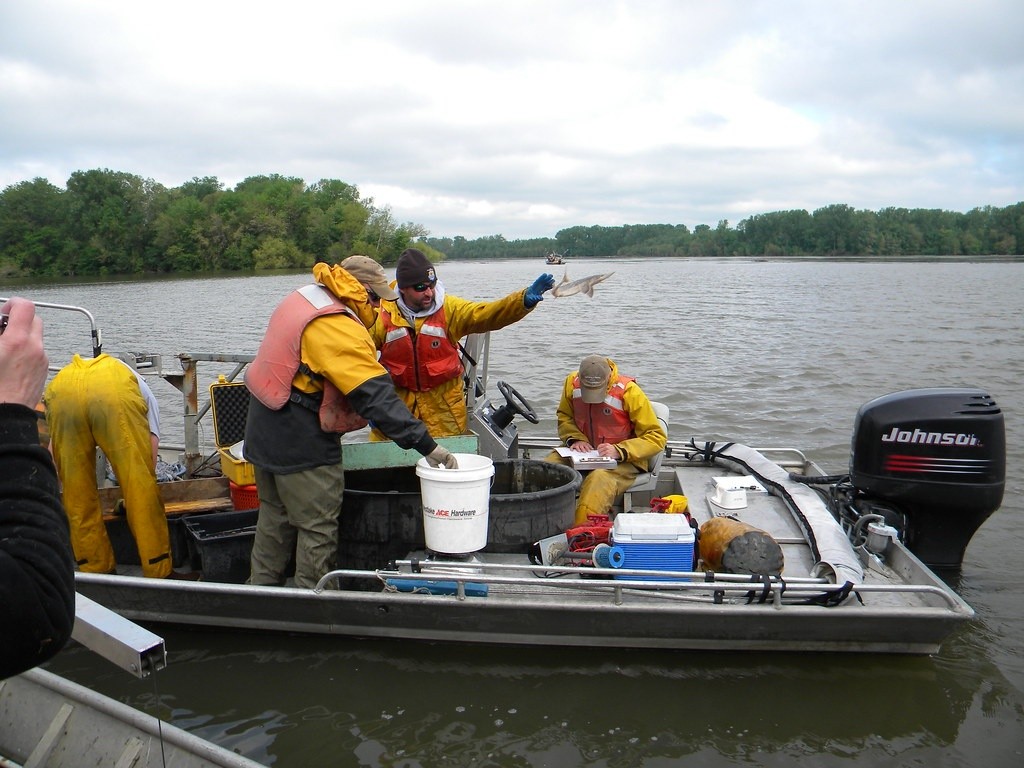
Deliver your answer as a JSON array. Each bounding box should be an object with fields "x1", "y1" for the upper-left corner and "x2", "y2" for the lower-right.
[{"x1": 416, "y1": 453, "x2": 490, "y2": 554}]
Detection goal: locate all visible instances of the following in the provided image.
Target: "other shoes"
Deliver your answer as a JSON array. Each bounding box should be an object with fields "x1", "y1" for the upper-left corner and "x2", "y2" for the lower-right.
[{"x1": 165, "y1": 570, "x2": 202, "y2": 581}]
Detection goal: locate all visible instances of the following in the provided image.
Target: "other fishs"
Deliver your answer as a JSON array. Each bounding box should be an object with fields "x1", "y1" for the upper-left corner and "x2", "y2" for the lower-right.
[{"x1": 551, "y1": 271, "x2": 618, "y2": 298}]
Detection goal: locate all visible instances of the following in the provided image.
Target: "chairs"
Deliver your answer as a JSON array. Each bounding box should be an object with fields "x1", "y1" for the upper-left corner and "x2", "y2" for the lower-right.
[{"x1": 623, "y1": 399, "x2": 670, "y2": 513}]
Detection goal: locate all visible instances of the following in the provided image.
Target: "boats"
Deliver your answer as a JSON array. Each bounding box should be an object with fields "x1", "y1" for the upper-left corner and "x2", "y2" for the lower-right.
[
  {"x1": 545, "y1": 252, "x2": 566, "y2": 264},
  {"x1": 0, "y1": 299, "x2": 1006, "y2": 655}
]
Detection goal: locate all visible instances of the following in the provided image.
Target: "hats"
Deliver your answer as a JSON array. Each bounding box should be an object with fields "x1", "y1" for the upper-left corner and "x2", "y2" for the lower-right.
[
  {"x1": 340, "y1": 255, "x2": 399, "y2": 302},
  {"x1": 578, "y1": 355, "x2": 610, "y2": 403},
  {"x1": 396, "y1": 248, "x2": 438, "y2": 288}
]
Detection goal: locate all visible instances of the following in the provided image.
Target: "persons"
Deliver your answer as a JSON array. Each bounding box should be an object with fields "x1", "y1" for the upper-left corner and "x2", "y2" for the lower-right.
[
  {"x1": 34, "y1": 354, "x2": 202, "y2": 582},
  {"x1": 535, "y1": 357, "x2": 667, "y2": 530},
  {"x1": 0, "y1": 297, "x2": 78, "y2": 683},
  {"x1": 247, "y1": 256, "x2": 458, "y2": 590},
  {"x1": 366, "y1": 248, "x2": 555, "y2": 444}
]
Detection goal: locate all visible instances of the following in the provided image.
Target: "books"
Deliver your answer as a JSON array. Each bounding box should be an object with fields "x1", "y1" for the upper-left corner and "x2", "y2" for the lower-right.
[{"x1": 554, "y1": 446, "x2": 616, "y2": 469}]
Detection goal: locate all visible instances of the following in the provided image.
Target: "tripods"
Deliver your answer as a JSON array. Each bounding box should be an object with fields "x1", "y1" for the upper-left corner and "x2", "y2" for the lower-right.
[{"x1": 378, "y1": 558, "x2": 830, "y2": 598}]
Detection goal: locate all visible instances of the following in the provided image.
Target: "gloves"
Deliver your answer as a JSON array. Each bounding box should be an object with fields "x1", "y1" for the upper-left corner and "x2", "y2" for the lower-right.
[
  {"x1": 524, "y1": 273, "x2": 555, "y2": 309},
  {"x1": 425, "y1": 445, "x2": 459, "y2": 470}
]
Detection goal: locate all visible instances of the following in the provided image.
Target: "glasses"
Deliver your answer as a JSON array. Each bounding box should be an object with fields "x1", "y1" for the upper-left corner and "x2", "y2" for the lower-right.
[
  {"x1": 411, "y1": 281, "x2": 437, "y2": 292},
  {"x1": 366, "y1": 288, "x2": 383, "y2": 302}
]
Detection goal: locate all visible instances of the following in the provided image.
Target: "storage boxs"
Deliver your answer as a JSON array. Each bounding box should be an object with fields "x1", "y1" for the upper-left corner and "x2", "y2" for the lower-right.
[
  {"x1": 209, "y1": 374, "x2": 258, "y2": 487},
  {"x1": 183, "y1": 508, "x2": 260, "y2": 584},
  {"x1": 608, "y1": 512, "x2": 696, "y2": 585},
  {"x1": 127, "y1": 513, "x2": 190, "y2": 569}
]
